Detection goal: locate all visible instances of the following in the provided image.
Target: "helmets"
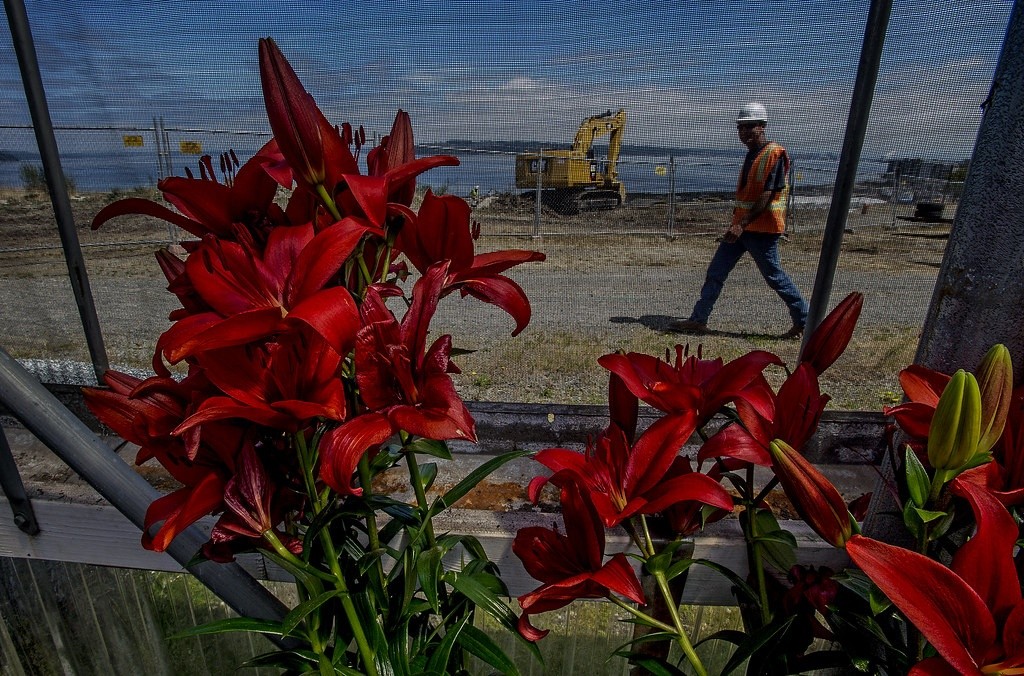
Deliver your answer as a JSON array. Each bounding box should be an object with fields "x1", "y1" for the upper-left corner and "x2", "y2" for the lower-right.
[{"x1": 735, "y1": 103, "x2": 767, "y2": 124}]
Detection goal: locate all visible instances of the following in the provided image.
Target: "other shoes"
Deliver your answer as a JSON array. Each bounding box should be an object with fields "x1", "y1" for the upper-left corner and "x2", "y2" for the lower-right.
[
  {"x1": 782, "y1": 325, "x2": 806, "y2": 340},
  {"x1": 669, "y1": 320, "x2": 707, "y2": 336}
]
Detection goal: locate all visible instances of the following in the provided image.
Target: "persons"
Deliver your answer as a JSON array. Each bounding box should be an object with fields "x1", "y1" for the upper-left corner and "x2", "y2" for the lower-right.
[{"x1": 669, "y1": 101, "x2": 809, "y2": 342}]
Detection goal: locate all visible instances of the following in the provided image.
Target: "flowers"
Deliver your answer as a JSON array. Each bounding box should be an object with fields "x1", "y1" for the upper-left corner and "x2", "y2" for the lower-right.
[{"x1": 89, "y1": 41, "x2": 1024, "y2": 676}]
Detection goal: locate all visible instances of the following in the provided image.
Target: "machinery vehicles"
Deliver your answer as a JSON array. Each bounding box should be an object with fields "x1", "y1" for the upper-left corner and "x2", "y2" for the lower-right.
[{"x1": 514, "y1": 106, "x2": 628, "y2": 214}]
